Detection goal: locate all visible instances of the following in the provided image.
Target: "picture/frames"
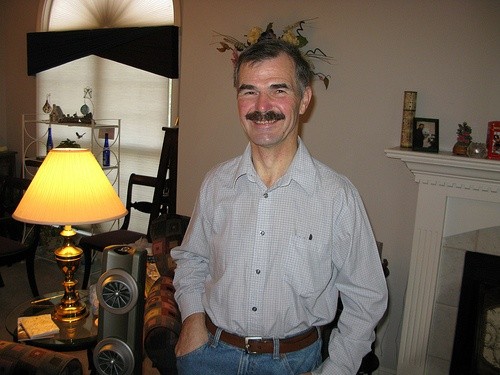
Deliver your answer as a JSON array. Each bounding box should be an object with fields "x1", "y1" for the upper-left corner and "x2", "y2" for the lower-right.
[
  {"x1": 486, "y1": 120, "x2": 500, "y2": 160},
  {"x1": 413, "y1": 118, "x2": 439, "y2": 154}
]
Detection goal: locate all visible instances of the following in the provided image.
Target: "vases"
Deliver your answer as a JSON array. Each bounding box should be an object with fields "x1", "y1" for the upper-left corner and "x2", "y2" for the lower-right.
[
  {"x1": 468, "y1": 143, "x2": 487, "y2": 159},
  {"x1": 454, "y1": 142, "x2": 469, "y2": 156}
]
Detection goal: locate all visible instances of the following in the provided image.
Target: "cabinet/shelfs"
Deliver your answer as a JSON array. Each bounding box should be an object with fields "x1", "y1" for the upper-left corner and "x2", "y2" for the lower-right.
[{"x1": 22, "y1": 114, "x2": 120, "y2": 276}]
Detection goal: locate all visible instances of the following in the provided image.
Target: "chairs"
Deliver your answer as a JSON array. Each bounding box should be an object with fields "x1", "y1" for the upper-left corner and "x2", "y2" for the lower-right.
[
  {"x1": 79, "y1": 174, "x2": 157, "y2": 290},
  {"x1": 145, "y1": 277, "x2": 181, "y2": 375},
  {"x1": 0, "y1": 177, "x2": 39, "y2": 297},
  {"x1": 0, "y1": 340, "x2": 83, "y2": 375}
]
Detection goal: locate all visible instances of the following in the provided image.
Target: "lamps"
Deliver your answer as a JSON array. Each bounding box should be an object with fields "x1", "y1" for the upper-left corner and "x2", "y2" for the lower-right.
[{"x1": 13, "y1": 149, "x2": 128, "y2": 320}]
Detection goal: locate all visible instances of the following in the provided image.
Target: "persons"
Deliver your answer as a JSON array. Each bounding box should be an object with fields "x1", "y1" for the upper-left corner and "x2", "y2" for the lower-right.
[{"x1": 170, "y1": 39, "x2": 389, "y2": 375}]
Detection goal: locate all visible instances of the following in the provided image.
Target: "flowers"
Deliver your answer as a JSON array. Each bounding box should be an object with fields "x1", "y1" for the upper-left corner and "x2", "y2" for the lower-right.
[
  {"x1": 216, "y1": 19, "x2": 331, "y2": 90},
  {"x1": 457, "y1": 121, "x2": 471, "y2": 142}
]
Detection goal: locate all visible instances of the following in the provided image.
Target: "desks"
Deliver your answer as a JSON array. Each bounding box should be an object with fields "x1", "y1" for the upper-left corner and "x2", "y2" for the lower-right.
[{"x1": 6, "y1": 290, "x2": 99, "y2": 375}]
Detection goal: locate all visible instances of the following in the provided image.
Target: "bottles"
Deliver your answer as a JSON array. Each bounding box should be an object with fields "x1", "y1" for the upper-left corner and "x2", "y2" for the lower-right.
[
  {"x1": 103, "y1": 133, "x2": 110, "y2": 166},
  {"x1": 467, "y1": 141, "x2": 489, "y2": 159},
  {"x1": 43, "y1": 100, "x2": 52, "y2": 113},
  {"x1": 47, "y1": 128, "x2": 53, "y2": 155},
  {"x1": 50, "y1": 104, "x2": 58, "y2": 122}
]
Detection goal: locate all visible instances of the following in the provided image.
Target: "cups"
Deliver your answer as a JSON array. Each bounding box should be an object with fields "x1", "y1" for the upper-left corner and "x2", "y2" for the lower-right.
[{"x1": 90, "y1": 283, "x2": 99, "y2": 315}]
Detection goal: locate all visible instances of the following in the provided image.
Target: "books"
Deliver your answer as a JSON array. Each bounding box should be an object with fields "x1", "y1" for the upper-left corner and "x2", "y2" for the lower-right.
[{"x1": 17, "y1": 314, "x2": 60, "y2": 341}]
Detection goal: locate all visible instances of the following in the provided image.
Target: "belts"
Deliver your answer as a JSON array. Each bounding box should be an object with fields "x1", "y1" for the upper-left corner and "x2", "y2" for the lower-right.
[{"x1": 205, "y1": 314, "x2": 323, "y2": 354}]
